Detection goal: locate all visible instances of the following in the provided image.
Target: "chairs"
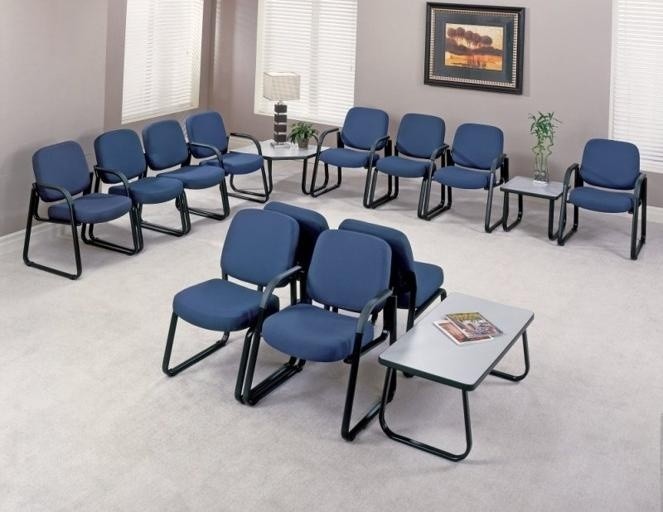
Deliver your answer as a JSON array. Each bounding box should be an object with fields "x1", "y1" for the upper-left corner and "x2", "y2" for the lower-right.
[
  {"x1": 142, "y1": 119, "x2": 226, "y2": 234},
  {"x1": 370, "y1": 113, "x2": 445, "y2": 218},
  {"x1": 558, "y1": 138, "x2": 648, "y2": 260},
  {"x1": 163, "y1": 208, "x2": 302, "y2": 405},
  {"x1": 242, "y1": 230, "x2": 398, "y2": 440},
  {"x1": 263, "y1": 202, "x2": 329, "y2": 304},
  {"x1": 93, "y1": 130, "x2": 187, "y2": 253},
  {"x1": 339, "y1": 219, "x2": 448, "y2": 377},
  {"x1": 23, "y1": 141, "x2": 138, "y2": 280},
  {"x1": 185, "y1": 112, "x2": 269, "y2": 217},
  {"x1": 423, "y1": 123, "x2": 509, "y2": 234},
  {"x1": 310, "y1": 107, "x2": 393, "y2": 208}
]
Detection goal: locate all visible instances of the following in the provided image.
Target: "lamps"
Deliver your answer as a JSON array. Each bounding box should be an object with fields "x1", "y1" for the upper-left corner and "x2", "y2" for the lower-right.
[{"x1": 263, "y1": 71, "x2": 300, "y2": 149}]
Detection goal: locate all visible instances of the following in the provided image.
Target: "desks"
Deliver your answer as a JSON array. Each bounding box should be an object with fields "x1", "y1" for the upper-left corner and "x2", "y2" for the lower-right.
[
  {"x1": 378, "y1": 293, "x2": 535, "y2": 461},
  {"x1": 229, "y1": 138, "x2": 332, "y2": 197},
  {"x1": 501, "y1": 176, "x2": 572, "y2": 241}
]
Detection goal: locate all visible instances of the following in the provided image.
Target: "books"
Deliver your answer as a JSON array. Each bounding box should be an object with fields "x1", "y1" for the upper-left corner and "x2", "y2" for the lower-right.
[{"x1": 432, "y1": 310, "x2": 503, "y2": 346}]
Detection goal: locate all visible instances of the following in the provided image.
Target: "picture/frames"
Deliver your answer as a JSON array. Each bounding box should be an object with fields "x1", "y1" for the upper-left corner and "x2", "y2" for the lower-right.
[{"x1": 424, "y1": 1, "x2": 525, "y2": 95}]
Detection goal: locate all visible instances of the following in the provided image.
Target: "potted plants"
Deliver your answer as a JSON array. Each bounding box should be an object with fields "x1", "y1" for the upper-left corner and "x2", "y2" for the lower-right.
[
  {"x1": 528, "y1": 109, "x2": 562, "y2": 186},
  {"x1": 287, "y1": 121, "x2": 319, "y2": 149}
]
